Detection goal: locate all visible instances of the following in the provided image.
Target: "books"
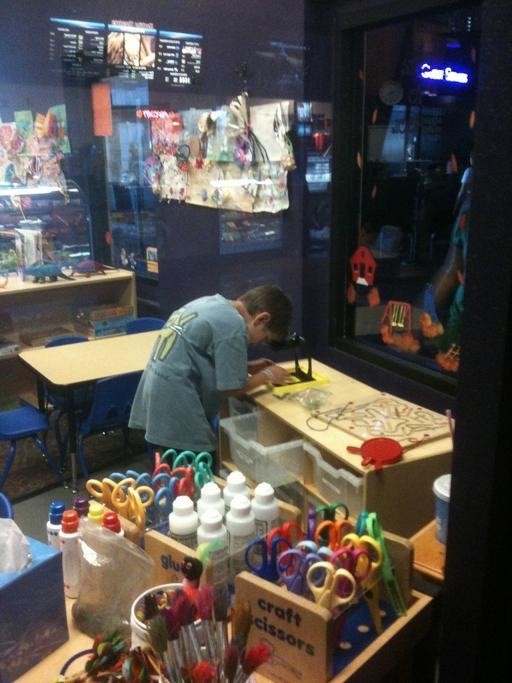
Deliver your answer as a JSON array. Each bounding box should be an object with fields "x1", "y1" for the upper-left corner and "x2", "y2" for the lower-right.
[
  {"x1": 19, "y1": 326, "x2": 75, "y2": 348},
  {"x1": 75, "y1": 315, "x2": 135, "y2": 330},
  {"x1": 12, "y1": 225, "x2": 44, "y2": 284},
  {"x1": 76, "y1": 302, "x2": 134, "y2": 319},
  {"x1": 0, "y1": 339, "x2": 19, "y2": 355},
  {"x1": 73, "y1": 323, "x2": 129, "y2": 338}
]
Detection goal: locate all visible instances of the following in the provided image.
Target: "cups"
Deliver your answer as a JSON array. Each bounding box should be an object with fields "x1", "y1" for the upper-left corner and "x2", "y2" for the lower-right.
[{"x1": 430, "y1": 471, "x2": 452, "y2": 540}]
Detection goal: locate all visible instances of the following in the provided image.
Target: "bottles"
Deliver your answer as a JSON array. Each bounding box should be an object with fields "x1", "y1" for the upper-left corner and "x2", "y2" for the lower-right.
[
  {"x1": 170, "y1": 469, "x2": 281, "y2": 593},
  {"x1": 45, "y1": 496, "x2": 126, "y2": 600}
]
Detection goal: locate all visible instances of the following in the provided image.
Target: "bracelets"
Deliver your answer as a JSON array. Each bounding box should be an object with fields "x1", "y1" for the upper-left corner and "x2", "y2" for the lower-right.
[{"x1": 261, "y1": 367, "x2": 275, "y2": 380}]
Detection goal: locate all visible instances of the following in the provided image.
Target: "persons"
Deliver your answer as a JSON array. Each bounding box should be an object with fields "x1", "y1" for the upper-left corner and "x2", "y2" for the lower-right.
[
  {"x1": 128, "y1": 287, "x2": 294, "y2": 475},
  {"x1": 428, "y1": 146, "x2": 476, "y2": 359}
]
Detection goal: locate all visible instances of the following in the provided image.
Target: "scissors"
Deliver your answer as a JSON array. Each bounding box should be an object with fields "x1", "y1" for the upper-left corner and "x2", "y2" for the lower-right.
[
  {"x1": 85, "y1": 447, "x2": 214, "y2": 529},
  {"x1": 247, "y1": 501, "x2": 408, "y2": 649}
]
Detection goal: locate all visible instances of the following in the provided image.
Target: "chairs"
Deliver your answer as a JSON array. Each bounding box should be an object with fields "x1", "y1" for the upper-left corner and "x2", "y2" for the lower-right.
[{"x1": 0, "y1": 316, "x2": 170, "y2": 500}]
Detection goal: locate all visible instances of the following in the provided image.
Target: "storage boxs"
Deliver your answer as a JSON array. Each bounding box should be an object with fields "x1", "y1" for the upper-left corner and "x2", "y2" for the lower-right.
[{"x1": 222, "y1": 402, "x2": 368, "y2": 517}]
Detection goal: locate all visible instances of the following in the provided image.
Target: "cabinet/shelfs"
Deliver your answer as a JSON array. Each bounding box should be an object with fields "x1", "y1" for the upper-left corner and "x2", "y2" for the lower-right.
[
  {"x1": 226, "y1": 354, "x2": 463, "y2": 551},
  {"x1": 1, "y1": 261, "x2": 140, "y2": 395}
]
